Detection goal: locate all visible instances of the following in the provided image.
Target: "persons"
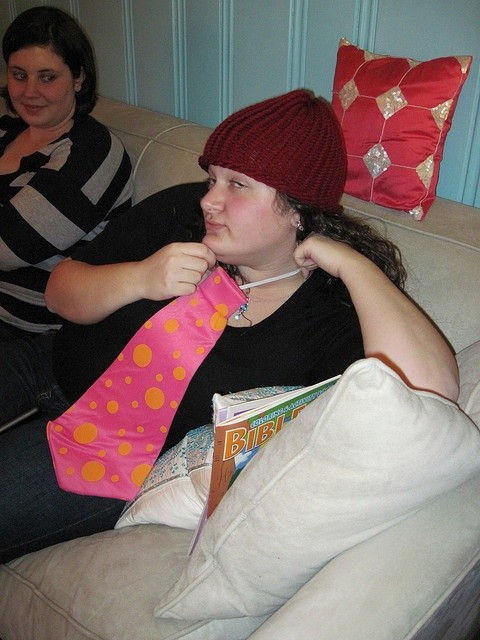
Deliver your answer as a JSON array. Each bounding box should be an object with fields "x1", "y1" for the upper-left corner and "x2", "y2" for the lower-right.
[
  {"x1": 0, "y1": 7, "x2": 135, "y2": 347},
  {"x1": 1, "y1": 91, "x2": 461, "y2": 566}
]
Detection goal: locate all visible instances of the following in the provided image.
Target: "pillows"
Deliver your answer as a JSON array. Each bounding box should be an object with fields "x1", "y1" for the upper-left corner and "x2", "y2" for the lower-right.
[
  {"x1": 113, "y1": 385, "x2": 309, "y2": 531},
  {"x1": 331, "y1": 37, "x2": 473, "y2": 221},
  {"x1": 151, "y1": 342, "x2": 479, "y2": 621}
]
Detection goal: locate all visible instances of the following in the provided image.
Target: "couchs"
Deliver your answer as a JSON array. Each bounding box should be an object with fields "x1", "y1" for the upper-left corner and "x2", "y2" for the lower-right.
[{"x1": 0, "y1": 94, "x2": 479, "y2": 639}]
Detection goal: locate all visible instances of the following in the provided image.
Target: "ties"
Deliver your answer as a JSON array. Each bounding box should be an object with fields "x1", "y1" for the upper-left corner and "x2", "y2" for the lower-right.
[{"x1": 45, "y1": 268, "x2": 311, "y2": 502}]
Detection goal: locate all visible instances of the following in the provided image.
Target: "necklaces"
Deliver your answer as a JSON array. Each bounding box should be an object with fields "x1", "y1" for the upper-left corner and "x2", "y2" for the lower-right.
[{"x1": 228, "y1": 266, "x2": 303, "y2": 321}]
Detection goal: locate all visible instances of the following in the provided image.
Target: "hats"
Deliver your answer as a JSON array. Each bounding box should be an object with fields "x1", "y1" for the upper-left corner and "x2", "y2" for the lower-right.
[{"x1": 199, "y1": 89, "x2": 348, "y2": 216}]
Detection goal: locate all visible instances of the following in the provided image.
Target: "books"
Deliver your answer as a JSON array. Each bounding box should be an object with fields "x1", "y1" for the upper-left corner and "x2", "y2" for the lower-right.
[{"x1": 189, "y1": 374, "x2": 343, "y2": 556}]
